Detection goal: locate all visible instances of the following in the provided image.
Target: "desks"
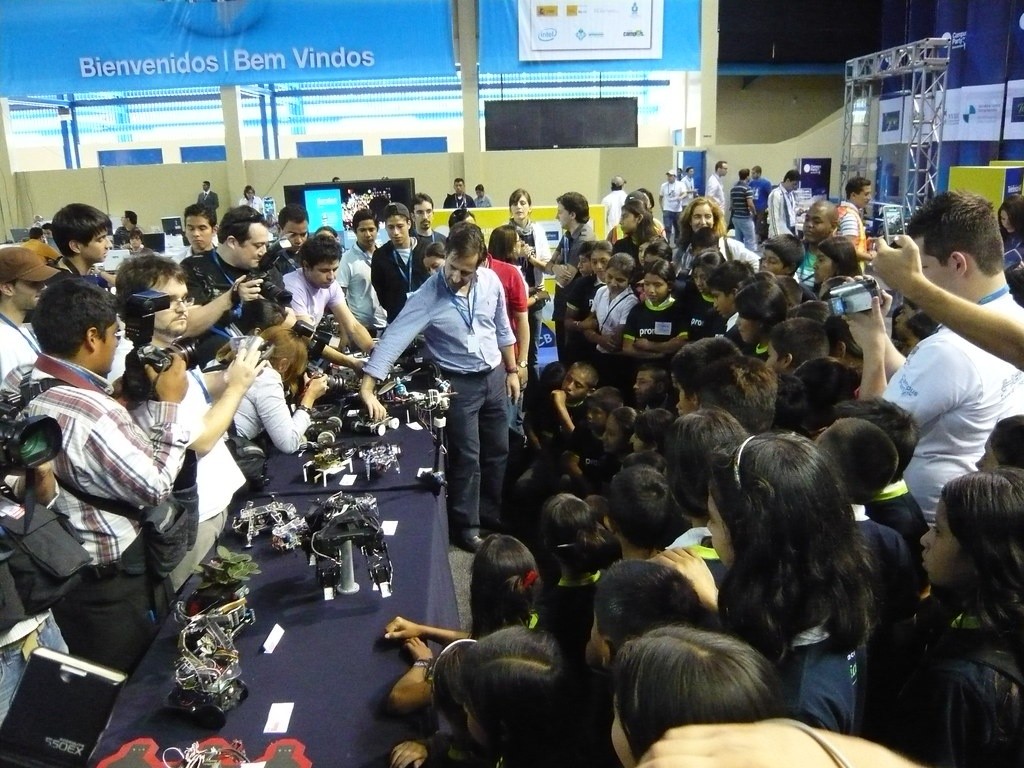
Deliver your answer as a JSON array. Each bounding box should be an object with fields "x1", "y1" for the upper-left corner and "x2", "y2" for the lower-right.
[
  {"x1": 244, "y1": 394, "x2": 450, "y2": 543},
  {"x1": 87, "y1": 492, "x2": 464, "y2": 768}
]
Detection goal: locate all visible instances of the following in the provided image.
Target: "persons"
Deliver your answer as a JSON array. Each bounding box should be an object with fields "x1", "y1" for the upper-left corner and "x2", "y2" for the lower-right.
[
  {"x1": 198, "y1": 181, "x2": 219, "y2": 212},
  {"x1": 772, "y1": 170, "x2": 803, "y2": 239},
  {"x1": 602, "y1": 177, "x2": 627, "y2": 230},
  {"x1": 332, "y1": 177, "x2": 341, "y2": 182},
  {"x1": 749, "y1": 166, "x2": 771, "y2": 238},
  {"x1": 730, "y1": 170, "x2": 756, "y2": 252},
  {"x1": 706, "y1": 161, "x2": 728, "y2": 212},
  {"x1": 659, "y1": 171, "x2": 687, "y2": 244},
  {"x1": 837, "y1": 178, "x2": 877, "y2": 273},
  {"x1": 443, "y1": 178, "x2": 476, "y2": 208},
  {"x1": 677, "y1": 167, "x2": 698, "y2": 211},
  {"x1": 473, "y1": 184, "x2": 492, "y2": 207},
  {"x1": 239, "y1": 184, "x2": 263, "y2": 213},
  {"x1": 0, "y1": 189, "x2": 1024, "y2": 768}
]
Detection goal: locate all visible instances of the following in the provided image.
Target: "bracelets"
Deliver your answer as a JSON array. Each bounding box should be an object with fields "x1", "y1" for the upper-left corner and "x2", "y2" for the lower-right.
[
  {"x1": 551, "y1": 264, "x2": 556, "y2": 274},
  {"x1": 533, "y1": 295, "x2": 538, "y2": 302}
]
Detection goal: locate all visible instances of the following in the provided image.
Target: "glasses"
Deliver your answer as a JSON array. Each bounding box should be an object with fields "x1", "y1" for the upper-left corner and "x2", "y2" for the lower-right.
[
  {"x1": 223, "y1": 213, "x2": 264, "y2": 230},
  {"x1": 516, "y1": 237, "x2": 521, "y2": 243},
  {"x1": 168, "y1": 297, "x2": 194, "y2": 310}
]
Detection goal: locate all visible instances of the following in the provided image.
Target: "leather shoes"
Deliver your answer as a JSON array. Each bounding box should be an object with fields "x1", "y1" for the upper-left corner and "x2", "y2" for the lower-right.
[
  {"x1": 480, "y1": 517, "x2": 516, "y2": 533},
  {"x1": 463, "y1": 536, "x2": 485, "y2": 551}
]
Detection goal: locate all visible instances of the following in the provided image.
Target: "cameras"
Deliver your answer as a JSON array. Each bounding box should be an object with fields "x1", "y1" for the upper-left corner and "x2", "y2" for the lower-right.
[
  {"x1": 821, "y1": 278, "x2": 885, "y2": 316},
  {"x1": 0, "y1": 396, "x2": 63, "y2": 483},
  {"x1": 242, "y1": 236, "x2": 347, "y2": 397},
  {"x1": 124, "y1": 291, "x2": 208, "y2": 401},
  {"x1": 882, "y1": 205, "x2": 904, "y2": 250}
]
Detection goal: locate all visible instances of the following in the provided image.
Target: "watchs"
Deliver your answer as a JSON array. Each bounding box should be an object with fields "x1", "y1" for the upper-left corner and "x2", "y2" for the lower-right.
[
  {"x1": 506, "y1": 367, "x2": 519, "y2": 374},
  {"x1": 517, "y1": 361, "x2": 528, "y2": 368}
]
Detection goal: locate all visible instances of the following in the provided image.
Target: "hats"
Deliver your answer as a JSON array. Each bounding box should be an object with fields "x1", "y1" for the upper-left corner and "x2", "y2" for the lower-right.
[
  {"x1": 0, "y1": 248, "x2": 61, "y2": 283},
  {"x1": 384, "y1": 202, "x2": 410, "y2": 219},
  {"x1": 666, "y1": 170, "x2": 677, "y2": 176},
  {"x1": 611, "y1": 177, "x2": 625, "y2": 186}
]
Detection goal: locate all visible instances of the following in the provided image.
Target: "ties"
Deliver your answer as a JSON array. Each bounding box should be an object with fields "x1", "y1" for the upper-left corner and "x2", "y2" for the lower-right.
[{"x1": 203, "y1": 193, "x2": 207, "y2": 200}]
